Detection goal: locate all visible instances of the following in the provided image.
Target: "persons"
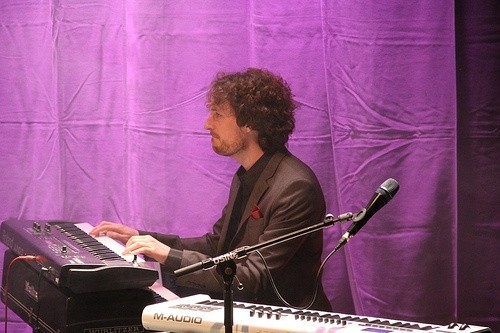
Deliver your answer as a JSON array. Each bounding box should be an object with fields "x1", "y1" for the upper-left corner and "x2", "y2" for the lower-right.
[{"x1": 88, "y1": 67, "x2": 339, "y2": 314}]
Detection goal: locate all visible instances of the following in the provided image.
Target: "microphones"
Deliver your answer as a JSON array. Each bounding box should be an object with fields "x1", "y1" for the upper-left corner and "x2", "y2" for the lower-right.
[{"x1": 335, "y1": 178, "x2": 400, "y2": 250}]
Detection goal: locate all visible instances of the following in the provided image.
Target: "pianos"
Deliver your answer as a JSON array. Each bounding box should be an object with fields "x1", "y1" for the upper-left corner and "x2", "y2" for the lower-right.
[
  {"x1": 0, "y1": 249, "x2": 181, "y2": 333},
  {"x1": 0, "y1": 219, "x2": 159, "y2": 294},
  {"x1": 141, "y1": 293, "x2": 493, "y2": 333}
]
